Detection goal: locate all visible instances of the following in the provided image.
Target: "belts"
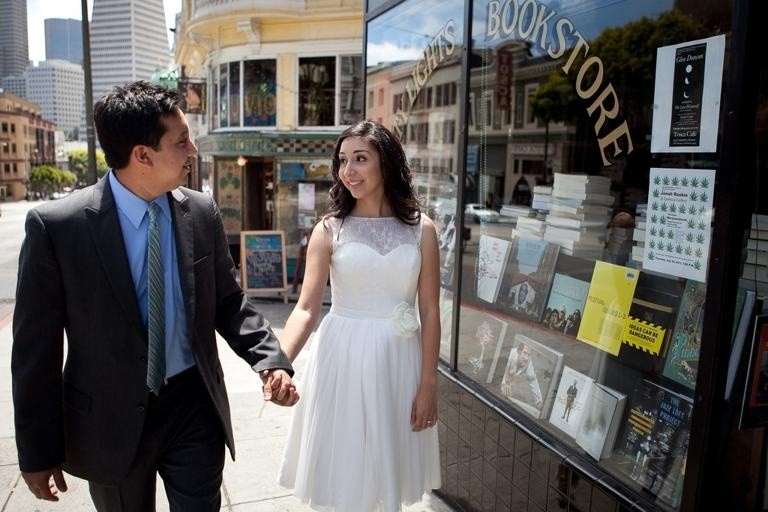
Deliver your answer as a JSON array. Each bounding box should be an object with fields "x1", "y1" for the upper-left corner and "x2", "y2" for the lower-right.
[{"x1": 144, "y1": 373, "x2": 196, "y2": 394}]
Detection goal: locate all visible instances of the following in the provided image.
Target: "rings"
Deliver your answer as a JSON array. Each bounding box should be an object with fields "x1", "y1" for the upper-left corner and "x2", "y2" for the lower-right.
[{"x1": 427, "y1": 420, "x2": 434, "y2": 425}]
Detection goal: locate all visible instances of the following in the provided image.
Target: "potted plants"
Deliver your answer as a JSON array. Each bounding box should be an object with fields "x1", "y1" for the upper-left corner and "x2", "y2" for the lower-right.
[{"x1": 28, "y1": 165, "x2": 58, "y2": 194}]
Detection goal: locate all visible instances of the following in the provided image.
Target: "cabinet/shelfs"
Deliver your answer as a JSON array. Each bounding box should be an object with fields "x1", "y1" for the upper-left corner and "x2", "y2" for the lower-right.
[{"x1": 416, "y1": 216, "x2": 706, "y2": 512}]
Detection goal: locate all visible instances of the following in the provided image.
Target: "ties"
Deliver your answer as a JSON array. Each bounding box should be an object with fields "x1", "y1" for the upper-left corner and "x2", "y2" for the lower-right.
[{"x1": 145, "y1": 205, "x2": 170, "y2": 395}]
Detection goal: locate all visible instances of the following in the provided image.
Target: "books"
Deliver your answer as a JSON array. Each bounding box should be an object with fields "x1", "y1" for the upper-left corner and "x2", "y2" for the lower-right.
[{"x1": 459, "y1": 171, "x2": 768, "y2": 509}]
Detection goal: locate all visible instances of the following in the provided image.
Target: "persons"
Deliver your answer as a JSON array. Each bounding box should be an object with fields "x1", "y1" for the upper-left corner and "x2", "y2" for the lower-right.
[
  {"x1": 11, "y1": 80, "x2": 300, "y2": 511},
  {"x1": 260, "y1": 122, "x2": 442, "y2": 512}
]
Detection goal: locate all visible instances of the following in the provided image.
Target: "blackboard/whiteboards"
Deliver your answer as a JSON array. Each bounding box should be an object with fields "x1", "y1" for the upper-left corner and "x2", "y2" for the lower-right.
[{"x1": 240, "y1": 230, "x2": 288, "y2": 292}]
